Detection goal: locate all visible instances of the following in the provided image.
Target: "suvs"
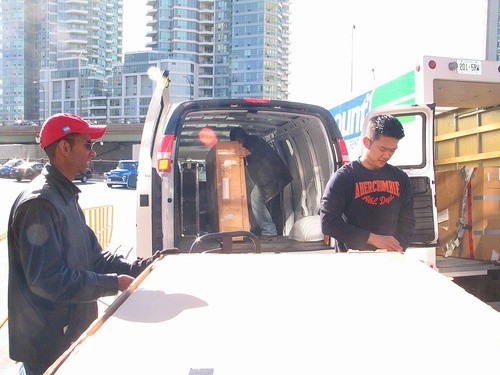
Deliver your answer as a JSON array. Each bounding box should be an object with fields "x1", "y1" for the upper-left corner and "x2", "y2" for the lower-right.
[{"x1": 104, "y1": 160, "x2": 138, "y2": 188}]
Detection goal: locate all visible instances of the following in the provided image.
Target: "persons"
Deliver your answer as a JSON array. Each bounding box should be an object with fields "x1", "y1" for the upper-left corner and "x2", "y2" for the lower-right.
[
  {"x1": 230, "y1": 127, "x2": 293, "y2": 237},
  {"x1": 320, "y1": 115, "x2": 416, "y2": 253},
  {"x1": 7, "y1": 112, "x2": 180, "y2": 375}
]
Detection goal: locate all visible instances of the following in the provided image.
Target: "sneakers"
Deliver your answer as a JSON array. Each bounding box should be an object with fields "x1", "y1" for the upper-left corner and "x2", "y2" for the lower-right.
[{"x1": 255, "y1": 235, "x2": 278, "y2": 242}]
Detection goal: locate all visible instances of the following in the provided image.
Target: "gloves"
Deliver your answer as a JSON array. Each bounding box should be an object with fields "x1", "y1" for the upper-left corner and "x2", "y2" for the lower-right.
[{"x1": 136, "y1": 248, "x2": 181, "y2": 273}]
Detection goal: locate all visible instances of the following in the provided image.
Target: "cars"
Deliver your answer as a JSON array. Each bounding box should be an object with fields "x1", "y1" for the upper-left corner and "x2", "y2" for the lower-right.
[
  {"x1": 0, "y1": 158, "x2": 24, "y2": 178},
  {"x1": 74, "y1": 167, "x2": 93, "y2": 183},
  {"x1": 9, "y1": 161, "x2": 44, "y2": 182}
]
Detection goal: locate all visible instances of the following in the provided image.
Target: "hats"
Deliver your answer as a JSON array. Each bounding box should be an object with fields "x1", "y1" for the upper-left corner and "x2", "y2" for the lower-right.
[{"x1": 39, "y1": 113, "x2": 107, "y2": 149}]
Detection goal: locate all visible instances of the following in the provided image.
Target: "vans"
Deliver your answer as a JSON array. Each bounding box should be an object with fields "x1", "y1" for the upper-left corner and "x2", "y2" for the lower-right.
[{"x1": 135, "y1": 71, "x2": 440, "y2": 258}]
